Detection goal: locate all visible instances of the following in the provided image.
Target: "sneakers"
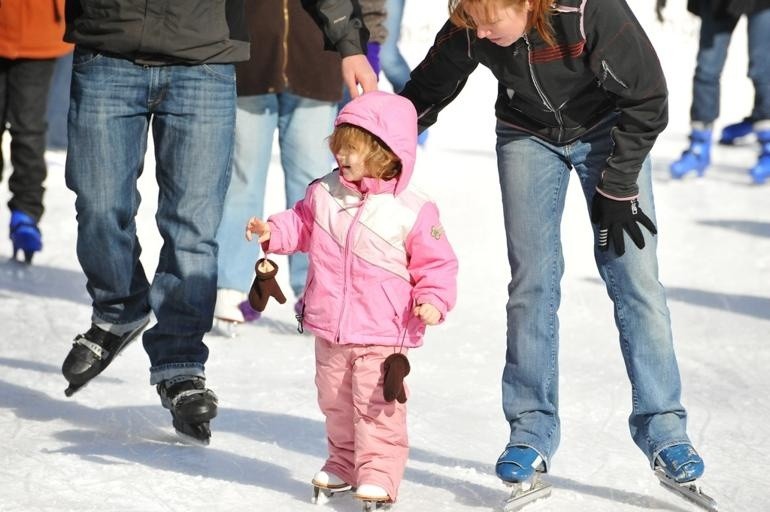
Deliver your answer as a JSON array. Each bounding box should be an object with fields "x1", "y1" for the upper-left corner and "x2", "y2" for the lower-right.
[
  {"x1": 59, "y1": 315, "x2": 151, "y2": 387},
  {"x1": 352, "y1": 482, "x2": 390, "y2": 502},
  {"x1": 154, "y1": 375, "x2": 218, "y2": 426},
  {"x1": 10, "y1": 210, "x2": 44, "y2": 252},
  {"x1": 495, "y1": 443, "x2": 544, "y2": 488},
  {"x1": 311, "y1": 469, "x2": 353, "y2": 493},
  {"x1": 654, "y1": 441, "x2": 705, "y2": 485},
  {"x1": 213, "y1": 287, "x2": 249, "y2": 324},
  {"x1": 721, "y1": 116, "x2": 770, "y2": 146}
]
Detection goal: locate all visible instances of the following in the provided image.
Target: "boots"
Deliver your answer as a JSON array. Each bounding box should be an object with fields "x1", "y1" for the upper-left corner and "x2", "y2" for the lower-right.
[
  {"x1": 748, "y1": 129, "x2": 770, "y2": 181},
  {"x1": 671, "y1": 131, "x2": 713, "y2": 180}
]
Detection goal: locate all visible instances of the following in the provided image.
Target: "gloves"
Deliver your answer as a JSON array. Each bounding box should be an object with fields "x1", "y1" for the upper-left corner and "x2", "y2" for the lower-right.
[
  {"x1": 247, "y1": 257, "x2": 287, "y2": 313},
  {"x1": 382, "y1": 352, "x2": 411, "y2": 404},
  {"x1": 590, "y1": 188, "x2": 658, "y2": 257}
]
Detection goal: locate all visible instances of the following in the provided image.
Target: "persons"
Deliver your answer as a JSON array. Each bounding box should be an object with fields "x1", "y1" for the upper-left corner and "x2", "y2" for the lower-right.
[
  {"x1": 653, "y1": 0, "x2": 769, "y2": 189},
  {"x1": 211, "y1": 0, "x2": 387, "y2": 340},
  {"x1": 61, "y1": 0, "x2": 251, "y2": 426},
  {"x1": 397, "y1": 0, "x2": 730, "y2": 488},
  {"x1": 0, "y1": 0, "x2": 74, "y2": 267},
  {"x1": 327, "y1": 0, "x2": 429, "y2": 159},
  {"x1": 246, "y1": 90, "x2": 459, "y2": 512}
]
